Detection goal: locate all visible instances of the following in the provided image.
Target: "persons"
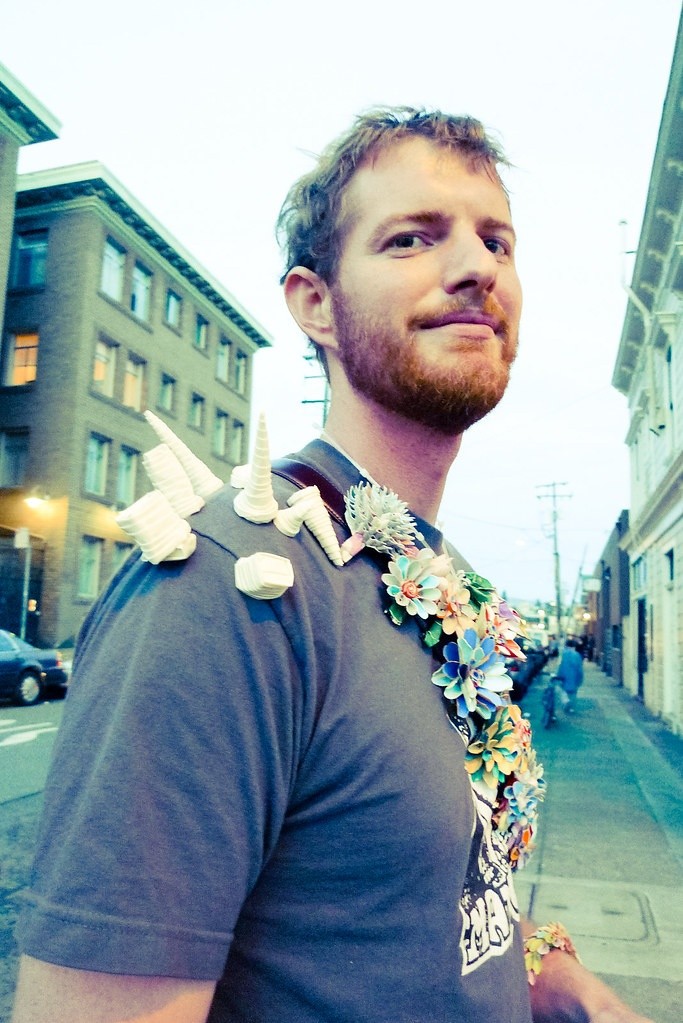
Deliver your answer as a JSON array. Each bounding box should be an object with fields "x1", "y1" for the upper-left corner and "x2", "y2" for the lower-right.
[
  {"x1": 11, "y1": 108, "x2": 653, "y2": 1023},
  {"x1": 579, "y1": 634, "x2": 595, "y2": 661},
  {"x1": 555, "y1": 640, "x2": 583, "y2": 713}
]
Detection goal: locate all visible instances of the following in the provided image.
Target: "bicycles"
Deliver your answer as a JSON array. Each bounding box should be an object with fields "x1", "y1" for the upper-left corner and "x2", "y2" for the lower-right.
[{"x1": 539, "y1": 672, "x2": 564, "y2": 730}]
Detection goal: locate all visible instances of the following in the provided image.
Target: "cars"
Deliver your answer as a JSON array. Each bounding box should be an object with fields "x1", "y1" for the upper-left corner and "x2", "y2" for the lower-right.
[
  {"x1": 504, "y1": 638, "x2": 545, "y2": 698},
  {"x1": 0, "y1": 629, "x2": 69, "y2": 707}
]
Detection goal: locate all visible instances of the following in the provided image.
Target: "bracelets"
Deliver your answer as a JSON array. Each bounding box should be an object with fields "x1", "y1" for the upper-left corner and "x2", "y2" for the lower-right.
[{"x1": 523, "y1": 922, "x2": 583, "y2": 986}]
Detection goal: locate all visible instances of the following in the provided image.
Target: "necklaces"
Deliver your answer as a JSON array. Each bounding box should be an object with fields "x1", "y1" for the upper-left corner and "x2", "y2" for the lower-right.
[{"x1": 344, "y1": 481, "x2": 548, "y2": 873}]
